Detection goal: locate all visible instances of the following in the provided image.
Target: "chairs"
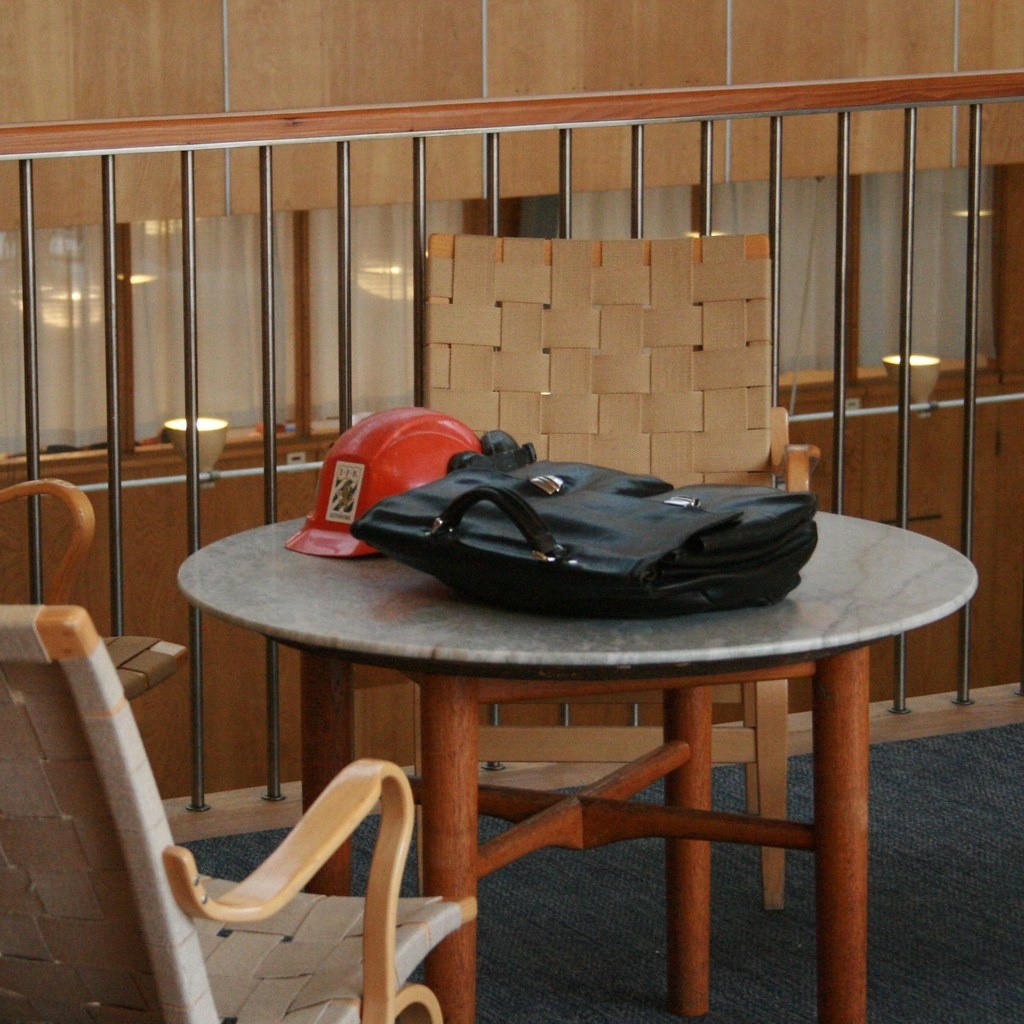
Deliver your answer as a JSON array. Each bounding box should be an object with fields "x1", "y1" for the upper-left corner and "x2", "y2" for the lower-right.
[
  {"x1": 423, "y1": 231, "x2": 822, "y2": 910},
  {"x1": 0, "y1": 606, "x2": 478, "y2": 1024},
  {"x1": 0, "y1": 477, "x2": 191, "y2": 700}
]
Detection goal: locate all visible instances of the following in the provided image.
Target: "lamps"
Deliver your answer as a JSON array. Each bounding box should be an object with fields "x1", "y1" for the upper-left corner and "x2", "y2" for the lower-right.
[
  {"x1": 881, "y1": 353, "x2": 942, "y2": 418},
  {"x1": 165, "y1": 417, "x2": 231, "y2": 489}
]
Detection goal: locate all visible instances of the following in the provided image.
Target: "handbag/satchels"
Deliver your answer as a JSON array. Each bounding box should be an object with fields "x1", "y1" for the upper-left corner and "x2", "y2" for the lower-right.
[{"x1": 350, "y1": 459, "x2": 817, "y2": 619}]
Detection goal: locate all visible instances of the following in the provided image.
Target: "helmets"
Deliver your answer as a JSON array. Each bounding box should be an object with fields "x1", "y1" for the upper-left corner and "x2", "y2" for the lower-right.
[{"x1": 285, "y1": 406, "x2": 537, "y2": 557}]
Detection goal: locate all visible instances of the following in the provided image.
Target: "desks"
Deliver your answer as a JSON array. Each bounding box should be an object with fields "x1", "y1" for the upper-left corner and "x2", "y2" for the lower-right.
[{"x1": 178, "y1": 511, "x2": 979, "y2": 1024}]
